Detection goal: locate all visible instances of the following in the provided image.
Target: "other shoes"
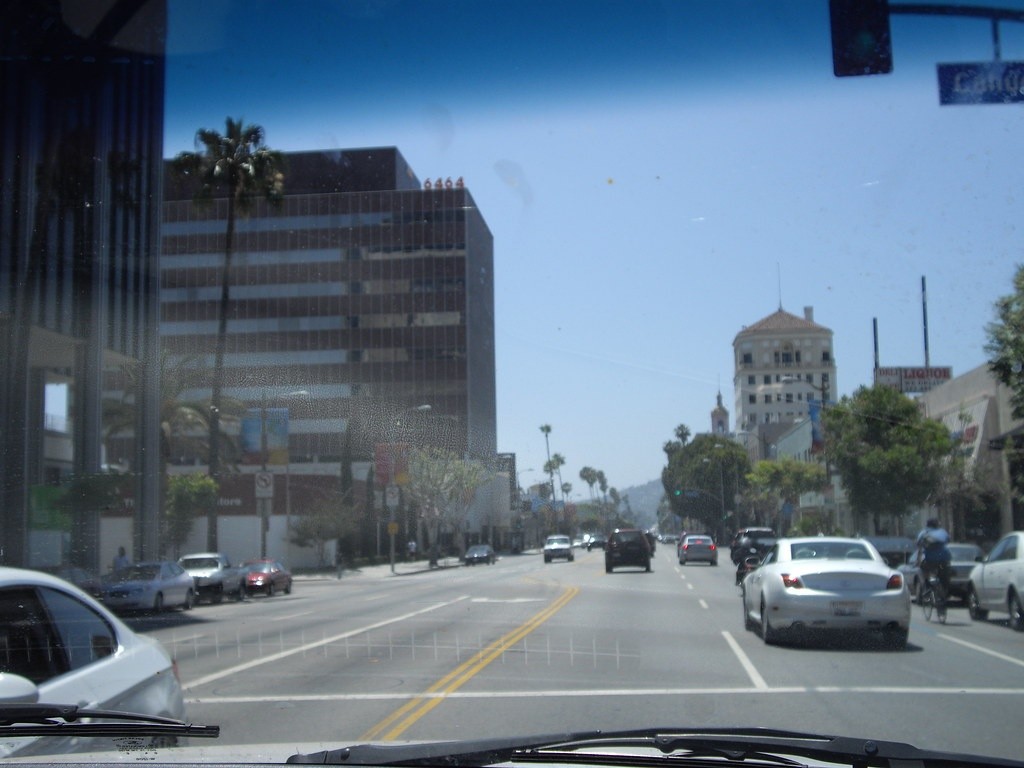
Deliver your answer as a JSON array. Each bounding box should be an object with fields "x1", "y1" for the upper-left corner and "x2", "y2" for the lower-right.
[{"x1": 922, "y1": 579, "x2": 930, "y2": 594}]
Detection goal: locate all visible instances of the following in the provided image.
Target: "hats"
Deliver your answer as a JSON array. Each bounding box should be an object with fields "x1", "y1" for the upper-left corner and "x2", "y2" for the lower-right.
[{"x1": 927, "y1": 518, "x2": 938, "y2": 525}]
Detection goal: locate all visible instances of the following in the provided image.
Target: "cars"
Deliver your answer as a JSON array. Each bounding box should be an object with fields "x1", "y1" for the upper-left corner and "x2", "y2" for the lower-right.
[
  {"x1": 102, "y1": 560, "x2": 196, "y2": 615},
  {"x1": 676, "y1": 530, "x2": 705, "y2": 555},
  {"x1": 238, "y1": 559, "x2": 293, "y2": 597},
  {"x1": 572, "y1": 534, "x2": 606, "y2": 552},
  {"x1": 37, "y1": 562, "x2": 108, "y2": 603},
  {"x1": 896, "y1": 541, "x2": 987, "y2": 605},
  {"x1": 678, "y1": 534, "x2": 718, "y2": 566},
  {"x1": 644, "y1": 529, "x2": 680, "y2": 557},
  {"x1": 735, "y1": 532, "x2": 915, "y2": 647},
  {"x1": 0, "y1": 566, "x2": 186, "y2": 760},
  {"x1": 968, "y1": 530, "x2": 1024, "y2": 631},
  {"x1": 463, "y1": 544, "x2": 496, "y2": 566}
]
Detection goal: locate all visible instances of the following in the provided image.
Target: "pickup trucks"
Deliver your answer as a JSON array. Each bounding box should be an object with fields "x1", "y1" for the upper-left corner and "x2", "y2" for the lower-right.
[{"x1": 177, "y1": 553, "x2": 248, "y2": 604}]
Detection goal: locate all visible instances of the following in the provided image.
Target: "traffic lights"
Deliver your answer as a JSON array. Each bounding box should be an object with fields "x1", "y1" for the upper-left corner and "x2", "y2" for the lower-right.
[{"x1": 830, "y1": 1, "x2": 893, "y2": 76}]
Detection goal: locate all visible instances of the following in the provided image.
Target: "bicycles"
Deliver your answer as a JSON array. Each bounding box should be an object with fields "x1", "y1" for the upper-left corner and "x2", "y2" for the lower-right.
[{"x1": 911, "y1": 562, "x2": 947, "y2": 623}]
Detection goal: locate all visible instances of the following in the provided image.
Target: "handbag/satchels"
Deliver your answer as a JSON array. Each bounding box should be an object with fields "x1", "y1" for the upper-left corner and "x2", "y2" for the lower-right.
[{"x1": 922, "y1": 536, "x2": 940, "y2": 551}]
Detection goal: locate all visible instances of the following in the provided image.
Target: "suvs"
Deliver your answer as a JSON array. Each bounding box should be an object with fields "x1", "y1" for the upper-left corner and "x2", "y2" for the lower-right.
[
  {"x1": 543, "y1": 535, "x2": 575, "y2": 562},
  {"x1": 605, "y1": 529, "x2": 652, "y2": 572},
  {"x1": 730, "y1": 526, "x2": 776, "y2": 565}
]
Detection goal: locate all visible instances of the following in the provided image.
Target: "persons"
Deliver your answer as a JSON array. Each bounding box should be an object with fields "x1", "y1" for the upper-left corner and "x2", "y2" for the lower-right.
[
  {"x1": 113, "y1": 547, "x2": 129, "y2": 572},
  {"x1": 912, "y1": 517, "x2": 952, "y2": 602},
  {"x1": 408, "y1": 538, "x2": 417, "y2": 560}
]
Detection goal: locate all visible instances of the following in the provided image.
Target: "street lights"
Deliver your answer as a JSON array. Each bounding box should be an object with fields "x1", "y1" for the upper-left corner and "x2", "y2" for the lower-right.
[
  {"x1": 260, "y1": 387, "x2": 310, "y2": 560},
  {"x1": 713, "y1": 443, "x2": 740, "y2": 529},
  {"x1": 702, "y1": 458, "x2": 727, "y2": 546},
  {"x1": 389, "y1": 403, "x2": 432, "y2": 574}
]
